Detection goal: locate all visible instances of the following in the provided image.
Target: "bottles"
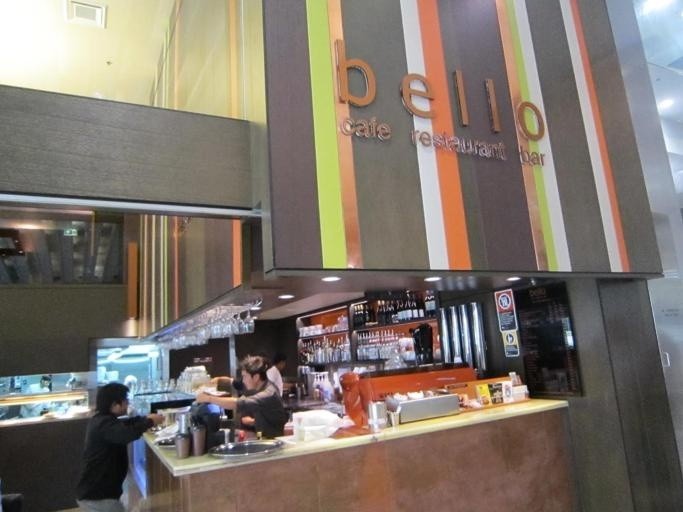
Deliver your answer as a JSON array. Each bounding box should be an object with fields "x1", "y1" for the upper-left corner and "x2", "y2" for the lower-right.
[
  {"x1": 300, "y1": 336, "x2": 351, "y2": 365},
  {"x1": 356, "y1": 329, "x2": 404, "y2": 360},
  {"x1": 311, "y1": 372, "x2": 342, "y2": 402},
  {"x1": 423, "y1": 290, "x2": 436, "y2": 317},
  {"x1": 353, "y1": 288, "x2": 426, "y2": 327}
]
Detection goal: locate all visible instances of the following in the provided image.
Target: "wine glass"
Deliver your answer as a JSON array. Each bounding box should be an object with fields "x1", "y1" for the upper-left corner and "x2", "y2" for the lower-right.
[
  {"x1": 156, "y1": 307, "x2": 257, "y2": 350},
  {"x1": 130, "y1": 377, "x2": 176, "y2": 395}
]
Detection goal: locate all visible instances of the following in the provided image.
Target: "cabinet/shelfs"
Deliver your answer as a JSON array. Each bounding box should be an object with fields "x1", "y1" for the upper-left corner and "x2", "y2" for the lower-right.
[{"x1": 295, "y1": 298, "x2": 444, "y2": 374}]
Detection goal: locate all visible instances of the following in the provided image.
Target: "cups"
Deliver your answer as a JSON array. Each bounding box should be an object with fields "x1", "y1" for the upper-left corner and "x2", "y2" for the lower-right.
[
  {"x1": 300, "y1": 316, "x2": 349, "y2": 336},
  {"x1": 176, "y1": 434, "x2": 188, "y2": 459},
  {"x1": 190, "y1": 425, "x2": 204, "y2": 457},
  {"x1": 177, "y1": 413, "x2": 189, "y2": 434}
]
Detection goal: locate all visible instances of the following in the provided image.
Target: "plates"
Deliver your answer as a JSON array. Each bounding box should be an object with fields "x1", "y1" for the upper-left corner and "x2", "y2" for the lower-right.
[{"x1": 98, "y1": 367, "x2": 120, "y2": 384}]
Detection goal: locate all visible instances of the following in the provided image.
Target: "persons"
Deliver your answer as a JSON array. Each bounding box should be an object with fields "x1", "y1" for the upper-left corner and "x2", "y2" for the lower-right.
[
  {"x1": 72, "y1": 381, "x2": 165, "y2": 512},
  {"x1": 265, "y1": 354, "x2": 287, "y2": 397},
  {"x1": 19, "y1": 375, "x2": 51, "y2": 417},
  {"x1": 194, "y1": 354, "x2": 287, "y2": 439}
]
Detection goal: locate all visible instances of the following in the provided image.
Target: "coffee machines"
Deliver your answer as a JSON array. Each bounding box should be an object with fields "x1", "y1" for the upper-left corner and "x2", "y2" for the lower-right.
[{"x1": 296, "y1": 366, "x2": 316, "y2": 401}]
